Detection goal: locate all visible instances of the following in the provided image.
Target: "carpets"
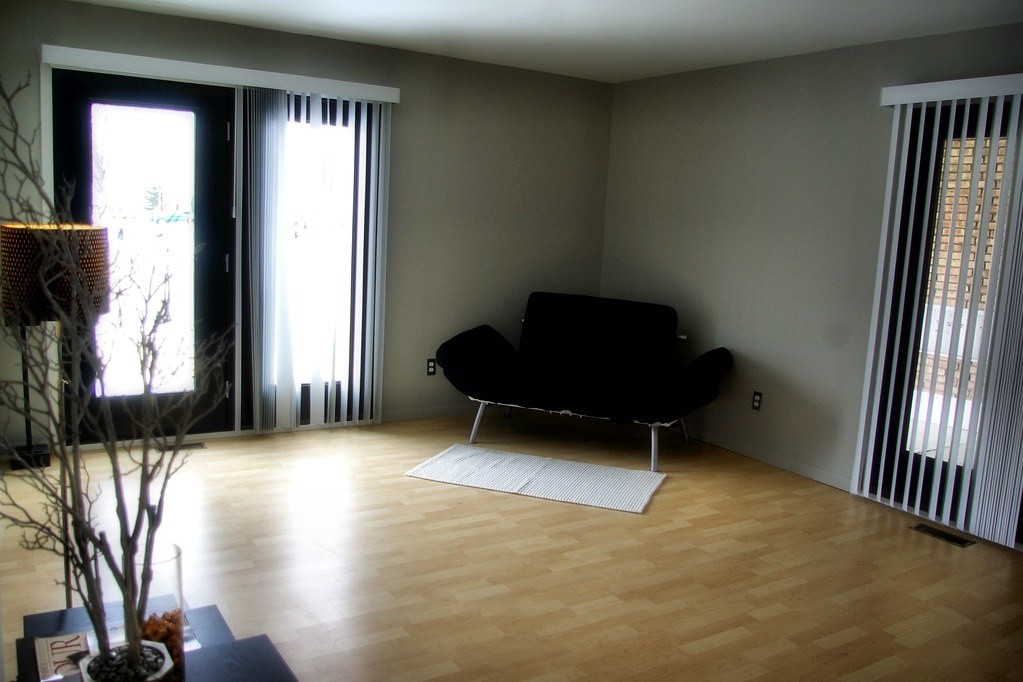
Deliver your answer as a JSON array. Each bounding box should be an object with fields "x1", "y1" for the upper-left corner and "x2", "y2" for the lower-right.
[{"x1": 405, "y1": 444, "x2": 667, "y2": 514}]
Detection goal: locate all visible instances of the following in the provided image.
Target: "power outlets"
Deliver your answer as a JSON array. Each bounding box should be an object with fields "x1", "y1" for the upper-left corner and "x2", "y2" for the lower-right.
[
  {"x1": 751, "y1": 392, "x2": 762, "y2": 409},
  {"x1": 427, "y1": 358, "x2": 436, "y2": 375}
]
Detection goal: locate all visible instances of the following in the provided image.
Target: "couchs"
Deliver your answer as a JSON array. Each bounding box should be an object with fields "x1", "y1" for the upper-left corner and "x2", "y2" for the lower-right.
[{"x1": 435, "y1": 291, "x2": 732, "y2": 472}]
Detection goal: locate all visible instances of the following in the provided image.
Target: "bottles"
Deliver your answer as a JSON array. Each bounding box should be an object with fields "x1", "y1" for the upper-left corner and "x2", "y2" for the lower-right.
[{"x1": 123, "y1": 543, "x2": 184, "y2": 680}]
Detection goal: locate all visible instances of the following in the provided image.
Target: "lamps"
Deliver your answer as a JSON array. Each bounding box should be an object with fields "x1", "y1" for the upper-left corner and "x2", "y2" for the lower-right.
[{"x1": 0, "y1": 223, "x2": 110, "y2": 471}]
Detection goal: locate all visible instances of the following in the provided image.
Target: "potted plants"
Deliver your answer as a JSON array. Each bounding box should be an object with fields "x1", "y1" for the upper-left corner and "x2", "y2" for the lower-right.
[{"x1": 0, "y1": 69, "x2": 175, "y2": 682}]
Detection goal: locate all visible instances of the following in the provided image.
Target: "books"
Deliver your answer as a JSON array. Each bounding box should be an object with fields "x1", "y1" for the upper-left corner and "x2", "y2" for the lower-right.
[{"x1": 35, "y1": 613, "x2": 201, "y2": 682}]
